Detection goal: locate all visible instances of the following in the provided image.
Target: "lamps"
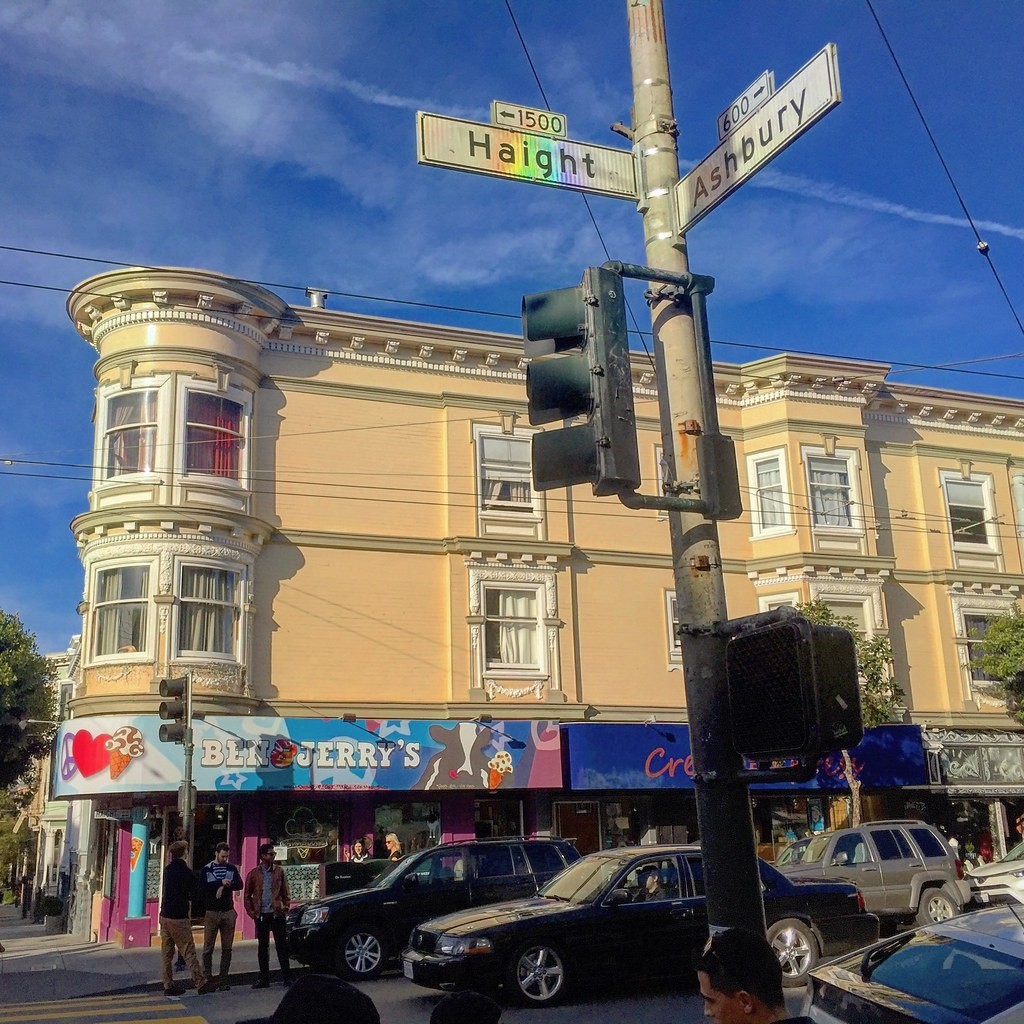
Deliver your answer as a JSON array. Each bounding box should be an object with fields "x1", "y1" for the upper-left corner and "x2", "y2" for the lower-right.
[
  {"x1": 469, "y1": 713, "x2": 492, "y2": 723},
  {"x1": 645, "y1": 715, "x2": 656, "y2": 728},
  {"x1": 584, "y1": 705, "x2": 601, "y2": 722},
  {"x1": 18, "y1": 719, "x2": 63, "y2": 730},
  {"x1": 339, "y1": 713, "x2": 356, "y2": 722}
]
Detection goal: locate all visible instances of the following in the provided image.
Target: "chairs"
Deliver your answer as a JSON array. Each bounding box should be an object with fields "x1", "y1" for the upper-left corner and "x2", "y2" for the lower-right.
[
  {"x1": 852, "y1": 843, "x2": 867, "y2": 863},
  {"x1": 454, "y1": 860, "x2": 464, "y2": 881},
  {"x1": 662, "y1": 869, "x2": 669, "y2": 883}
]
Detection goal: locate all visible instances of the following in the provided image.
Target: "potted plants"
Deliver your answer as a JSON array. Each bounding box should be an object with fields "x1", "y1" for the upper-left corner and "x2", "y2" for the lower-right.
[{"x1": 32, "y1": 895, "x2": 64, "y2": 933}]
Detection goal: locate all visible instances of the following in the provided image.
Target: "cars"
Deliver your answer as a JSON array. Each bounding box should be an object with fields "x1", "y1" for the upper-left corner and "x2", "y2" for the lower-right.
[
  {"x1": 400, "y1": 844, "x2": 881, "y2": 1009},
  {"x1": 798, "y1": 903, "x2": 1024, "y2": 1024}
]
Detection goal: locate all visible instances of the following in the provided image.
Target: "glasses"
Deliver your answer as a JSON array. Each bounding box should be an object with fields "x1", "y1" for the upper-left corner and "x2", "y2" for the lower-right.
[
  {"x1": 385, "y1": 840, "x2": 395, "y2": 845},
  {"x1": 701, "y1": 930, "x2": 727, "y2": 977},
  {"x1": 263, "y1": 851, "x2": 277, "y2": 857},
  {"x1": 638, "y1": 874, "x2": 654, "y2": 881}
]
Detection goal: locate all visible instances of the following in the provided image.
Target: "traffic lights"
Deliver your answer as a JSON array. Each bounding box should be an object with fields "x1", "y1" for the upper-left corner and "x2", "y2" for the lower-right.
[
  {"x1": 159, "y1": 676, "x2": 187, "y2": 745},
  {"x1": 521, "y1": 264, "x2": 642, "y2": 496},
  {"x1": 724, "y1": 617, "x2": 864, "y2": 762}
]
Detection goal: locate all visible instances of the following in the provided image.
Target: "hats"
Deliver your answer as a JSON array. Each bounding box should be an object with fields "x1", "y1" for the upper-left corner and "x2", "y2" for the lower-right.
[{"x1": 170, "y1": 841, "x2": 188, "y2": 853}]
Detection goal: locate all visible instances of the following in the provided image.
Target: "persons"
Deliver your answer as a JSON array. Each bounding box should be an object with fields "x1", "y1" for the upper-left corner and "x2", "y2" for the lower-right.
[
  {"x1": 159, "y1": 841, "x2": 219, "y2": 997},
  {"x1": 200, "y1": 842, "x2": 244, "y2": 989},
  {"x1": 344, "y1": 838, "x2": 373, "y2": 862},
  {"x1": 244, "y1": 844, "x2": 294, "y2": 988},
  {"x1": 269, "y1": 974, "x2": 381, "y2": 1024},
  {"x1": 697, "y1": 928, "x2": 817, "y2": 1024},
  {"x1": 36, "y1": 887, "x2": 42, "y2": 900},
  {"x1": 629, "y1": 866, "x2": 672, "y2": 899},
  {"x1": 430, "y1": 990, "x2": 502, "y2": 1024},
  {"x1": 385, "y1": 833, "x2": 401, "y2": 860}
]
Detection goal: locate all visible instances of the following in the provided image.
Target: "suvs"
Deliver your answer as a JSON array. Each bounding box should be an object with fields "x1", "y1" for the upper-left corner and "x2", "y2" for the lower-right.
[
  {"x1": 964, "y1": 841, "x2": 1024, "y2": 909},
  {"x1": 769, "y1": 819, "x2": 972, "y2": 930},
  {"x1": 285, "y1": 834, "x2": 582, "y2": 983}
]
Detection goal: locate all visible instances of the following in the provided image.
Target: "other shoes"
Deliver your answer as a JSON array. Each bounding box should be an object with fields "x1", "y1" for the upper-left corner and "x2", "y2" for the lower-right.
[
  {"x1": 283, "y1": 977, "x2": 292, "y2": 987},
  {"x1": 252, "y1": 978, "x2": 270, "y2": 989},
  {"x1": 164, "y1": 985, "x2": 186, "y2": 996},
  {"x1": 197, "y1": 980, "x2": 223, "y2": 995},
  {"x1": 219, "y1": 975, "x2": 230, "y2": 991}
]
